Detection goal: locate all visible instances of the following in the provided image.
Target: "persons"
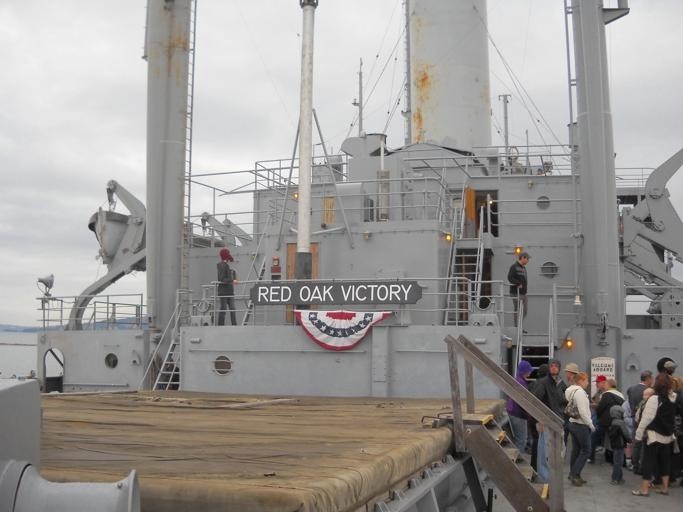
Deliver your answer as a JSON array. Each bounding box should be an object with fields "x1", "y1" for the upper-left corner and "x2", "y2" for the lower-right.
[
  {"x1": 216, "y1": 247, "x2": 239, "y2": 326},
  {"x1": 506, "y1": 252, "x2": 533, "y2": 334},
  {"x1": 502, "y1": 357, "x2": 682, "y2": 498}
]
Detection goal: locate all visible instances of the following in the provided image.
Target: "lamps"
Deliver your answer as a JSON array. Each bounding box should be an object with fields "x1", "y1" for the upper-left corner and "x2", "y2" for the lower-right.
[
  {"x1": 361, "y1": 230, "x2": 372, "y2": 240},
  {"x1": 513, "y1": 245, "x2": 523, "y2": 254},
  {"x1": 527, "y1": 179, "x2": 533, "y2": 188},
  {"x1": 442, "y1": 231, "x2": 451, "y2": 241},
  {"x1": 564, "y1": 336, "x2": 574, "y2": 349},
  {"x1": 293, "y1": 191, "x2": 299, "y2": 198}
]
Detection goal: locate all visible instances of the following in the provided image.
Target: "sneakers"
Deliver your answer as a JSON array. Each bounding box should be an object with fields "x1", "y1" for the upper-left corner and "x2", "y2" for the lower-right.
[
  {"x1": 568, "y1": 475, "x2": 587, "y2": 486},
  {"x1": 609, "y1": 480, "x2": 625, "y2": 486}
]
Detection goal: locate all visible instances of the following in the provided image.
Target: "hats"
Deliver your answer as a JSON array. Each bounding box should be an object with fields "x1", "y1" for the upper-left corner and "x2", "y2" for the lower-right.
[
  {"x1": 664, "y1": 361, "x2": 678, "y2": 368},
  {"x1": 592, "y1": 376, "x2": 606, "y2": 382},
  {"x1": 563, "y1": 363, "x2": 579, "y2": 373},
  {"x1": 519, "y1": 251, "x2": 532, "y2": 258}
]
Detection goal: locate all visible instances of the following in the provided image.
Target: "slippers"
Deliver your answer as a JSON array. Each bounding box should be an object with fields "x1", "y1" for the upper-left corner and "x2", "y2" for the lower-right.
[{"x1": 632, "y1": 488, "x2": 668, "y2": 496}]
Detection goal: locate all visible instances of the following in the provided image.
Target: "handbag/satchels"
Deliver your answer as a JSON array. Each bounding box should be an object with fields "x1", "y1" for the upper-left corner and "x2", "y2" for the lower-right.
[{"x1": 564, "y1": 406, "x2": 580, "y2": 419}]
